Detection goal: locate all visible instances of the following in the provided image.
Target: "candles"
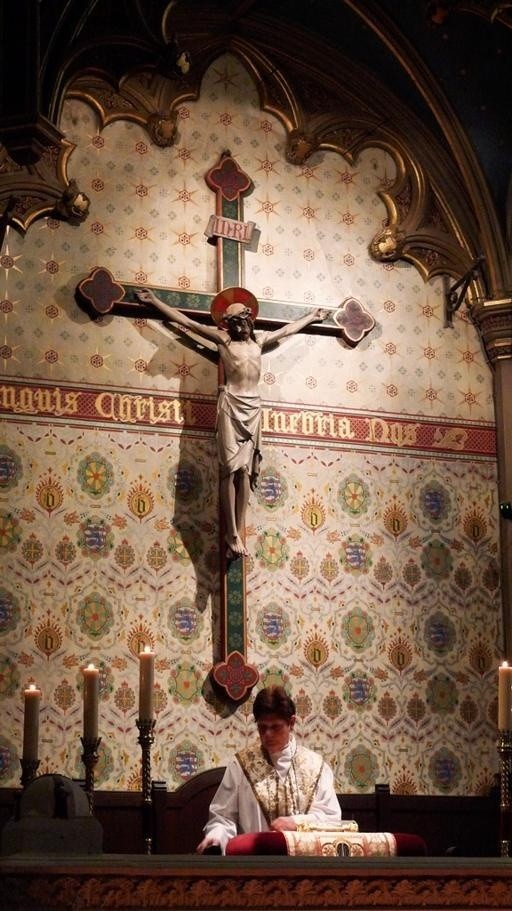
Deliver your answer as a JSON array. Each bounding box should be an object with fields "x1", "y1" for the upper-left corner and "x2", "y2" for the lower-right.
[
  {"x1": 83, "y1": 667, "x2": 100, "y2": 739},
  {"x1": 498, "y1": 666, "x2": 512, "y2": 732},
  {"x1": 23, "y1": 689, "x2": 40, "y2": 763},
  {"x1": 137, "y1": 651, "x2": 155, "y2": 723}
]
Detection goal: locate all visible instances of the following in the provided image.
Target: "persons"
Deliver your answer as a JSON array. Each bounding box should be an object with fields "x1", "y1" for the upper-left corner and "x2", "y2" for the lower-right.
[
  {"x1": 196, "y1": 684, "x2": 343, "y2": 856},
  {"x1": 134, "y1": 287, "x2": 332, "y2": 560}
]
